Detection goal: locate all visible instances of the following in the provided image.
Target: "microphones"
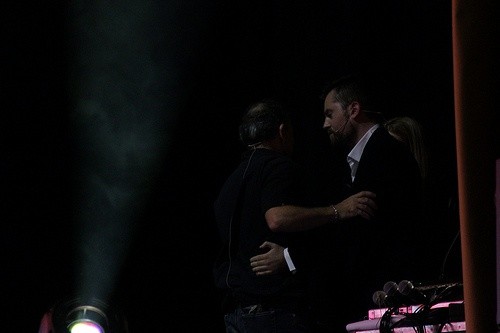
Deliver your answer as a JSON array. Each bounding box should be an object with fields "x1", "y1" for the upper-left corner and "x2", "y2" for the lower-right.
[
  {"x1": 373, "y1": 291, "x2": 393, "y2": 308},
  {"x1": 383, "y1": 282, "x2": 409, "y2": 307},
  {"x1": 398, "y1": 280, "x2": 429, "y2": 306},
  {"x1": 329, "y1": 114, "x2": 349, "y2": 144}
]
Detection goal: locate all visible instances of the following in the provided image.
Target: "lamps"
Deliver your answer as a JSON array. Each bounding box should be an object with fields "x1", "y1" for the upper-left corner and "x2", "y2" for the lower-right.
[{"x1": 50, "y1": 292, "x2": 129, "y2": 333}]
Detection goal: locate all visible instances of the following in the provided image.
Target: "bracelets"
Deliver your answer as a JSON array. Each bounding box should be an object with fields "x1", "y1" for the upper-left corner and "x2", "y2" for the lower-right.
[{"x1": 329, "y1": 204, "x2": 338, "y2": 221}]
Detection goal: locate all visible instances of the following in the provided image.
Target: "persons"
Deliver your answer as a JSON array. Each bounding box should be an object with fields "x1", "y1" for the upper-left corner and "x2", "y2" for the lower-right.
[
  {"x1": 249, "y1": 72, "x2": 423, "y2": 333},
  {"x1": 212, "y1": 100, "x2": 379, "y2": 333}
]
